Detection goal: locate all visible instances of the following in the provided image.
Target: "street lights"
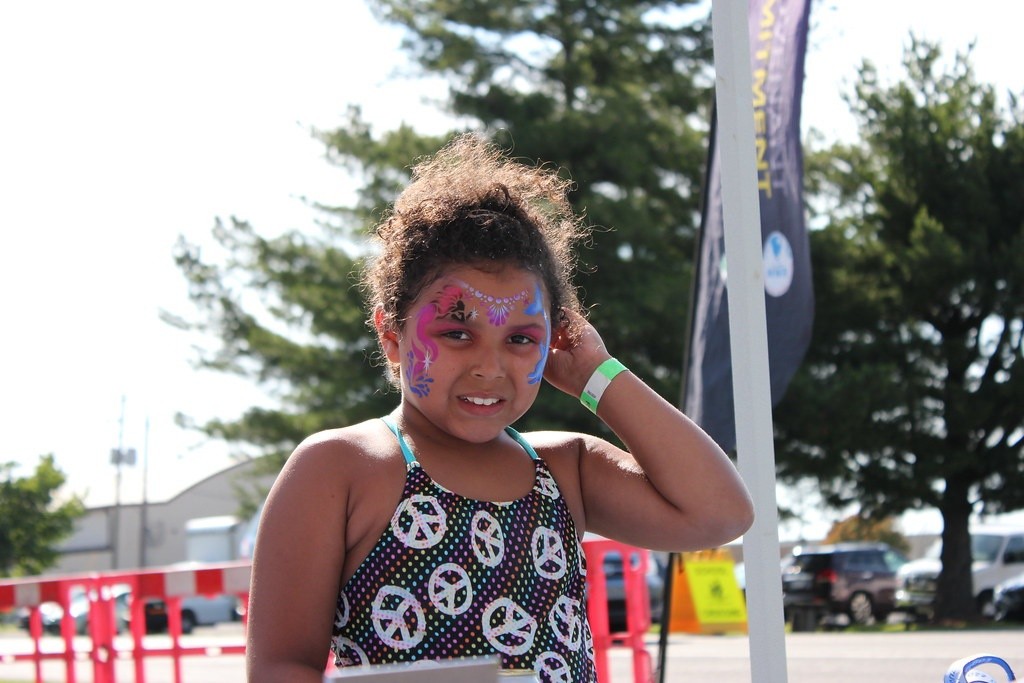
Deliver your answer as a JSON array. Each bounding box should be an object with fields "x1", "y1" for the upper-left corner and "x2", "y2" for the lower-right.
[{"x1": 110, "y1": 446, "x2": 136, "y2": 568}]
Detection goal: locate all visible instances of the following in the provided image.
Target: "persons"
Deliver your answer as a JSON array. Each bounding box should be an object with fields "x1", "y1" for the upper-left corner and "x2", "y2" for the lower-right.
[{"x1": 245, "y1": 128, "x2": 755, "y2": 683}]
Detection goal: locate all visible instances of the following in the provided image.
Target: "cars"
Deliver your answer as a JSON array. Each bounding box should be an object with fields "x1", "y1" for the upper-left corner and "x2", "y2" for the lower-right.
[
  {"x1": 781, "y1": 542, "x2": 895, "y2": 631},
  {"x1": 580, "y1": 532, "x2": 665, "y2": 645},
  {"x1": 892, "y1": 524, "x2": 1024, "y2": 619},
  {"x1": 18, "y1": 572, "x2": 132, "y2": 635},
  {"x1": 119, "y1": 562, "x2": 243, "y2": 635},
  {"x1": 993, "y1": 576, "x2": 1024, "y2": 625}
]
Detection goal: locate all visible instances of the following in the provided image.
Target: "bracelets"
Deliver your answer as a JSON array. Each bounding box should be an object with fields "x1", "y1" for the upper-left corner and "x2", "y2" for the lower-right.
[{"x1": 579, "y1": 356, "x2": 630, "y2": 416}]
